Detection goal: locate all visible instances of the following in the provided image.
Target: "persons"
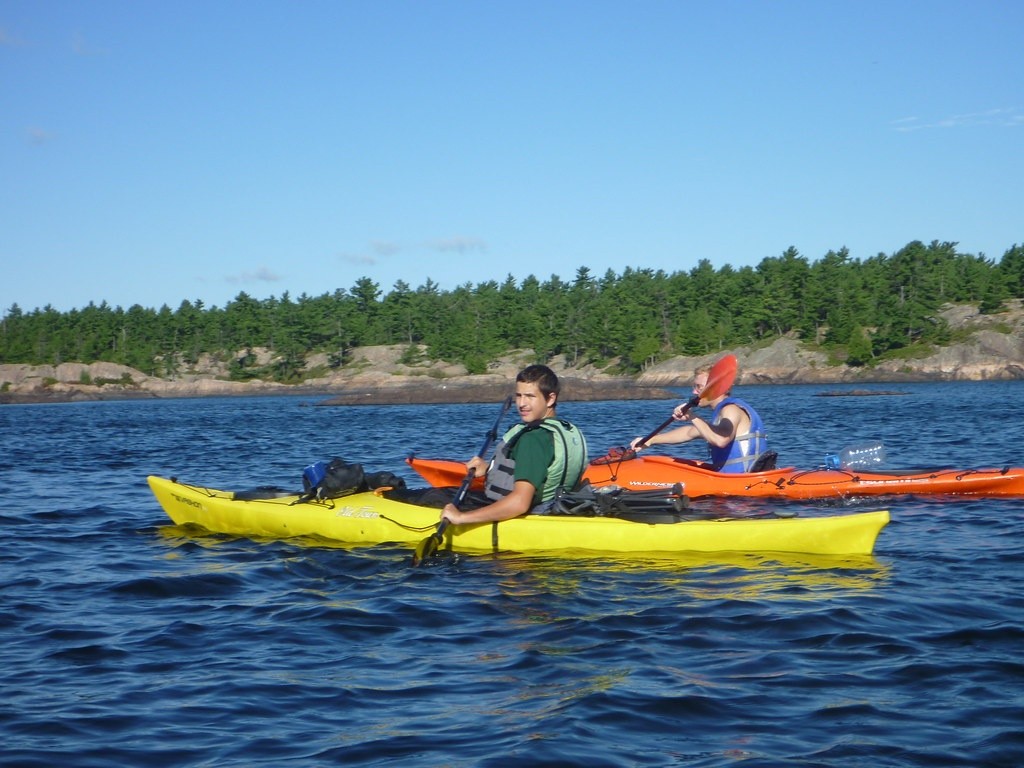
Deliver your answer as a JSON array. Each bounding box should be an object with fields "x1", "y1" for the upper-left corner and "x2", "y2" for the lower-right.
[
  {"x1": 379, "y1": 364, "x2": 589, "y2": 525},
  {"x1": 628, "y1": 362, "x2": 767, "y2": 474}
]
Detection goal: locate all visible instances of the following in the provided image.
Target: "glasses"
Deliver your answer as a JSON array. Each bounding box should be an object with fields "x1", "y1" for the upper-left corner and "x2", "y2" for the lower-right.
[{"x1": 691, "y1": 382, "x2": 705, "y2": 390}]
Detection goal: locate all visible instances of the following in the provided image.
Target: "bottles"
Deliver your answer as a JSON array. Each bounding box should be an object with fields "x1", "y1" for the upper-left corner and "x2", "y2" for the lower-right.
[{"x1": 825, "y1": 442, "x2": 884, "y2": 471}]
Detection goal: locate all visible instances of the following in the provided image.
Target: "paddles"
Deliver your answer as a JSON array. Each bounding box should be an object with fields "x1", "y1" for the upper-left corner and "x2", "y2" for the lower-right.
[
  {"x1": 636, "y1": 352, "x2": 739, "y2": 450},
  {"x1": 410, "y1": 395, "x2": 513, "y2": 560}
]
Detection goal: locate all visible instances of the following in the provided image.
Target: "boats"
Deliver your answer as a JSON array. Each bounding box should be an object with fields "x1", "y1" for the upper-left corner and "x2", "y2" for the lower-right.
[
  {"x1": 713, "y1": 499, "x2": 718, "y2": 516},
  {"x1": 401, "y1": 445, "x2": 1024, "y2": 511},
  {"x1": 142, "y1": 474, "x2": 896, "y2": 558}
]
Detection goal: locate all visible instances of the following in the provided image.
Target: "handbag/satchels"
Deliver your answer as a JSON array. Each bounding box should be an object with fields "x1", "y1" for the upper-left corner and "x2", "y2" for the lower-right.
[{"x1": 300, "y1": 456, "x2": 369, "y2": 500}]
